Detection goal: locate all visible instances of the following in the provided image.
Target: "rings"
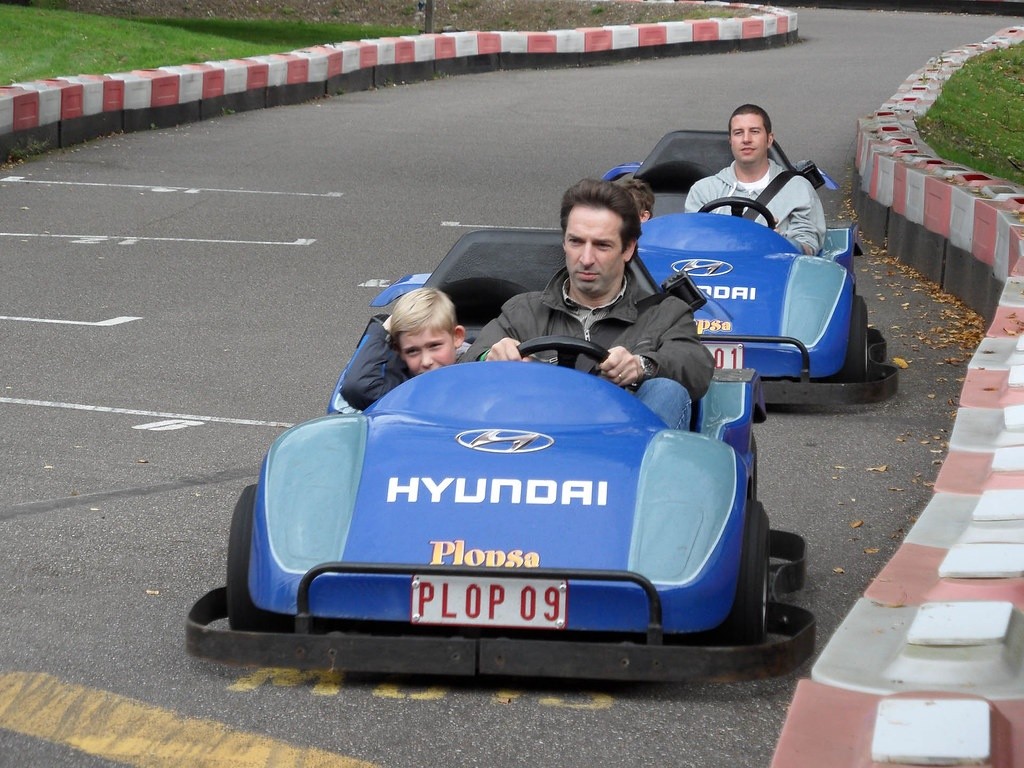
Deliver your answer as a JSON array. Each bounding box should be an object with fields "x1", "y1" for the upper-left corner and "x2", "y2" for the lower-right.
[{"x1": 619, "y1": 373, "x2": 622, "y2": 381}]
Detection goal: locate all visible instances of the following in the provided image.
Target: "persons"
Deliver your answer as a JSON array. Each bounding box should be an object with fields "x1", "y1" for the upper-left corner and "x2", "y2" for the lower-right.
[
  {"x1": 339, "y1": 287, "x2": 468, "y2": 411},
  {"x1": 685, "y1": 104, "x2": 826, "y2": 257},
  {"x1": 461, "y1": 179, "x2": 715, "y2": 432},
  {"x1": 616, "y1": 179, "x2": 655, "y2": 223}
]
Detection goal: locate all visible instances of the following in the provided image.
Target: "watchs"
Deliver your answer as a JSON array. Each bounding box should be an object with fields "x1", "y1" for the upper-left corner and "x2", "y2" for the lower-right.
[{"x1": 627, "y1": 355, "x2": 655, "y2": 391}]
